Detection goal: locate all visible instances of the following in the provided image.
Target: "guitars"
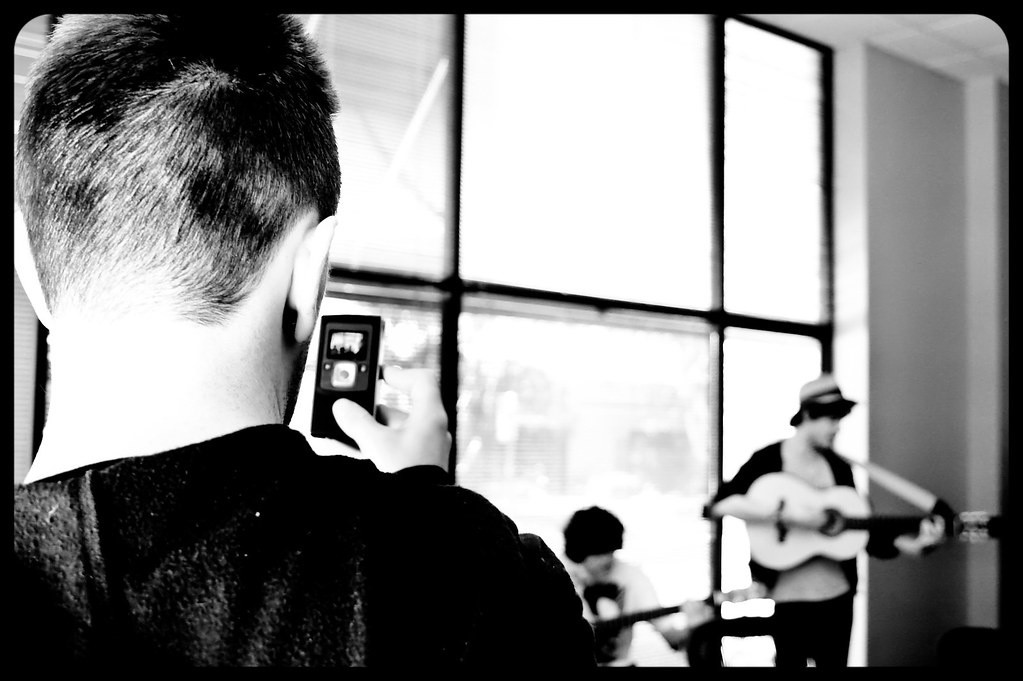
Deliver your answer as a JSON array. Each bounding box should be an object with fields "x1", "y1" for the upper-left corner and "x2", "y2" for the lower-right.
[
  {"x1": 742, "y1": 471, "x2": 1001, "y2": 572},
  {"x1": 582, "y1": 581, "x2": 768, "y2": 664}
]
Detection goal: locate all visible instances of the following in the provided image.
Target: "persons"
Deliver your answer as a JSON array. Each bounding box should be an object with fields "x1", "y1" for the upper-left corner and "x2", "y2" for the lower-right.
[
  {"x1": 558, "y1": 507, "x2": 714, "y2": 667},
  {"x1": 697, "y1": 372, "x2": 945, "y2": 668},
  {"x1": 7, "y1": 12, "x2": 602, "y2": 676}
]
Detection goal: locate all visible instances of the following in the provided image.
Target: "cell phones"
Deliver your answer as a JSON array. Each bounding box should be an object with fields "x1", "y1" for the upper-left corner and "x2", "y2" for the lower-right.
[{"x1": 310, "y1": 314, "x2": 386, "y2": 451}]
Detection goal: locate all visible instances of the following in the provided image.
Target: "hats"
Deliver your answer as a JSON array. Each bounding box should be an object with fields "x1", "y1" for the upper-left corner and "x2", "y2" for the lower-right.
[{"x1": 791, "y1": 375, "x2": 857, "y2": 425}]
